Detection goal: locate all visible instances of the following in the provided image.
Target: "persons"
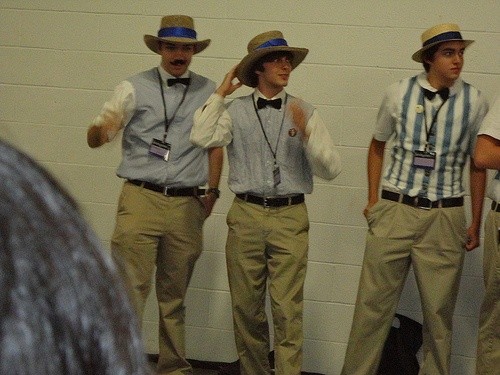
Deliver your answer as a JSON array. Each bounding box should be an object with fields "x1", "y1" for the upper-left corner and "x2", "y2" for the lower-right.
[
  {"x1": 189, "y1": 30, "x2": 343, "y2": 375},
  {"x1": 0, "y1": 140, "x2": 150, "y2": 375},
  {"x1": 474, "y1": 96, "x2": 500, "y2": 375},
  {"x1": 341, "y1": 23, "x2": 489, "y2": 375},
  {"x1": 86, "y1": 14, "x2": 223, "y2": 375}
]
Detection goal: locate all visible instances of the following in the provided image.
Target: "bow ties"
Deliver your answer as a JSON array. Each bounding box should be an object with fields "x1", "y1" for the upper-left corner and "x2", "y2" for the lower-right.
[
  {"x1": 168, "y1": 77, "x2": 191, "y2": 87},
  {"x1": 425, "y1": 87, "x2": 450, "y2": 100},
  {"x1": 257, "y1": 96, "x2": 283, "y2": 110}
]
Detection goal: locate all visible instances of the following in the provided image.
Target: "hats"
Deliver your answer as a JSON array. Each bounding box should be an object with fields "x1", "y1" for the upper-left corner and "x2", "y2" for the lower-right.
[
  {"x1": 236, "y1": 30, "x2": 309, "y2": 87},
  {"x1": 412, "y1": 22, "x2": 474, "y2": 63},
  {"x1": 144, "y1": 15, "x2": 211, "y2": 57}
]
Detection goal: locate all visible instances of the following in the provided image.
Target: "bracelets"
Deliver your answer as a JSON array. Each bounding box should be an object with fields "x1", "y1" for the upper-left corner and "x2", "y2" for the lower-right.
[{"x1": 208, "y1": 187, "x2": 220, "y2": 198}]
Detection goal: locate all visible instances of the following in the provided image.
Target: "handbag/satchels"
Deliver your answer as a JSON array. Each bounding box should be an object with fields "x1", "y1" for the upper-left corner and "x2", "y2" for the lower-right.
[{"x1": 378, "y1": 311, "x2": 424, "y2": 375}]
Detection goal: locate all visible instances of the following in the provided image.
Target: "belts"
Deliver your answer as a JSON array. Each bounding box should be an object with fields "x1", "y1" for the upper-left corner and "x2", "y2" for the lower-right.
[
  {"x1": 236, "y1": 193, "x2": 305, "y2": 209},
  {"x1": 490, "y1": 200, "x2": 500, "y2": 213},
  {"x1": 382, "y1": 189, "x2": 464, "y2": 210},
  {"x1": 128, "y1": 178, "x2": 201, "y2": 197}
]
